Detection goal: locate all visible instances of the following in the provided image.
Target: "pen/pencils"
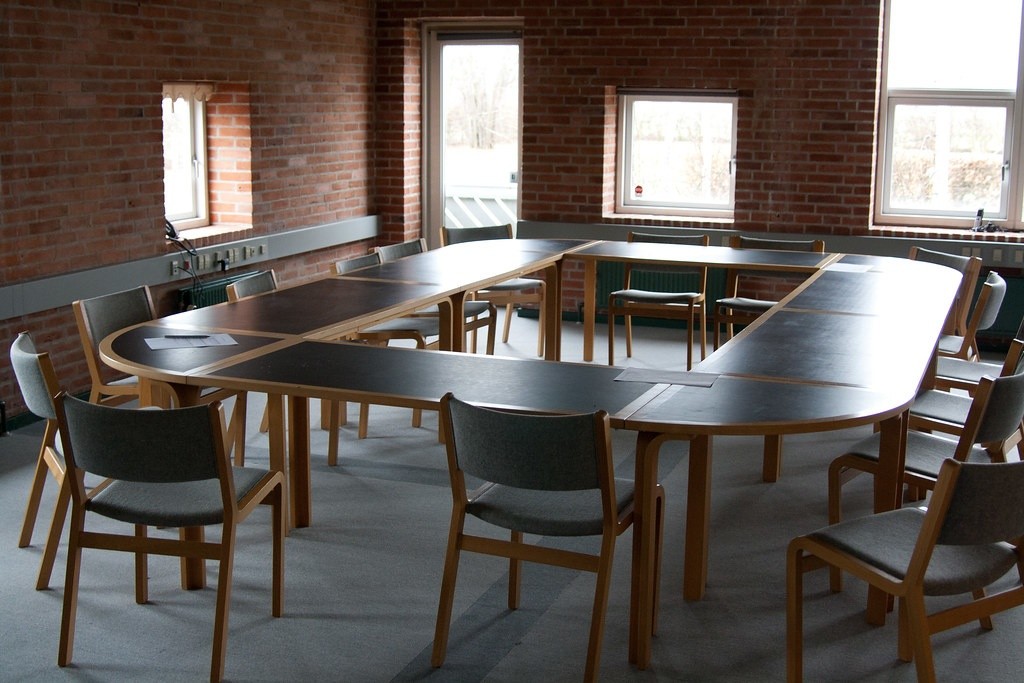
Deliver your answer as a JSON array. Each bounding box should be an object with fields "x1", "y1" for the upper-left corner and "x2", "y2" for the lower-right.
[{"x1": 165, "y1": 335, "x2": 211, "y2": 338}]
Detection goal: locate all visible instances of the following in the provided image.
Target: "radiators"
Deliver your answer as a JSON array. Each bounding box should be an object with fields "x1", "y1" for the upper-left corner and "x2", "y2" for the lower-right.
[{"x1": 595, "y1": 259, "x2": 728, "y2": 329}]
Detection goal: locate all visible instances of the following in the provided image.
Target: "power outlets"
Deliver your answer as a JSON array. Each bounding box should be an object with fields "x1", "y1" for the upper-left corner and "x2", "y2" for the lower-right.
[{"x1": 215, "y1": 252, "x2": 222, "y2": 266}]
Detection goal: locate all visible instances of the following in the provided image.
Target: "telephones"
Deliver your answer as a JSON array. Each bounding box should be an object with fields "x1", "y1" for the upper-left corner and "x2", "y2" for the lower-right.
[
  {"x1": 972, "y1": 208, "x2": 985, "y2": 232},
  {"x1": 165, "y1": 217, "x2": 179, "y2": 238}
]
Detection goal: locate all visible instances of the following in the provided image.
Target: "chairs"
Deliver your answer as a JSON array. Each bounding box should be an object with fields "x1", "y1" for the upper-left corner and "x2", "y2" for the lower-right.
[{"x1": 7, "y1": 223, "x2": 1024, "y2": 682}]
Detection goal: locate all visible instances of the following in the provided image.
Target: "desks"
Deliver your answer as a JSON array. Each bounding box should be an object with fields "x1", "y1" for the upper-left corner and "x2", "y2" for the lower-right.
[{"x1": 93, "y1": 239, "x2": 958, "y2": 669}]
